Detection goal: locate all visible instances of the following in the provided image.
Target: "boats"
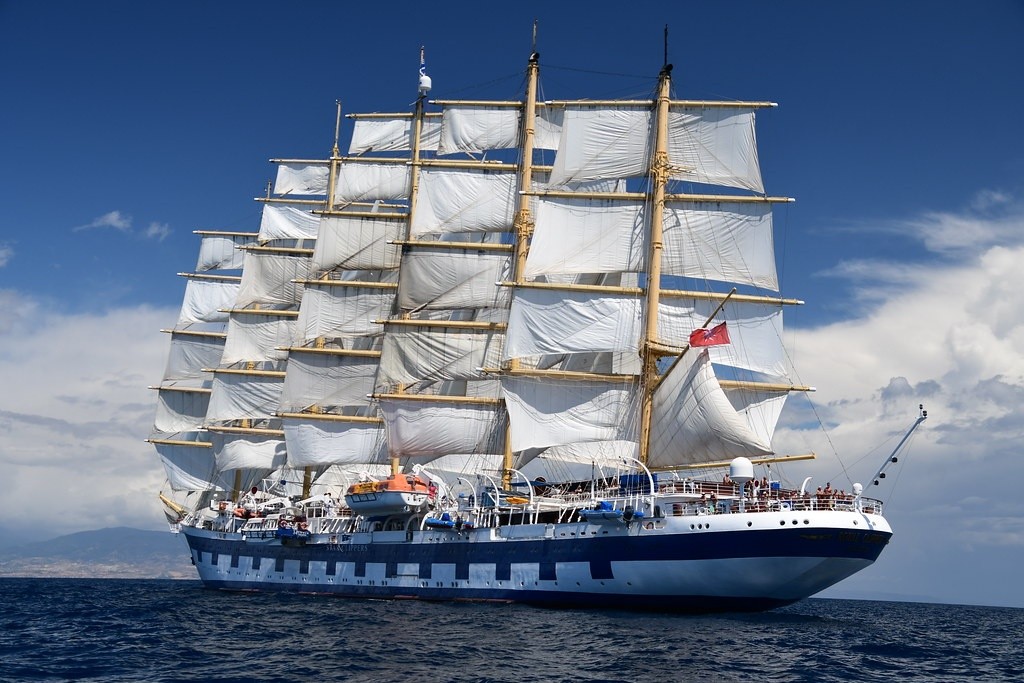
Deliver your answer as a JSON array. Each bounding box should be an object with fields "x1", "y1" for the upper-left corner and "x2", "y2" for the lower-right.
[
  {"x1": 577, "y1": 501, "x2": 645, "y2": 528},
  {"x1": 345, "y1": 474, "x2": 429, "y2": 516},
  {"x1": 425, "y1": 515, "x2": 477, "y2": 534},
  {"x1": 276, "y1": 525, "x2": 312, "y2": 541}
]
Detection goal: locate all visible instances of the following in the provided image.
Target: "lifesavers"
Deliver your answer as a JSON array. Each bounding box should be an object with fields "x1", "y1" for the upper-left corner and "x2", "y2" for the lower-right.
[
  {"x1": 280, "y1": 521, "x2": 287, "y2": 528},
  {"x1": 301, "y1": 522, "x2": 308, "y2": 530}
]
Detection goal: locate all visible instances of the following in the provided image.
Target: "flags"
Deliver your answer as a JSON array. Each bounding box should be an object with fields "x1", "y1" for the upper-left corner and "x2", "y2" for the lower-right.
[{"x1": 690, "y1": 320, "x2": 731, "y2": 347}]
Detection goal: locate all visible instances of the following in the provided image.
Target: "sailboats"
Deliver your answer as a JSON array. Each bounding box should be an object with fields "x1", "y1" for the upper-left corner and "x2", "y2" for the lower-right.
[{"x1": 141, "y1": 20, "x2": 931, "y2": 613}]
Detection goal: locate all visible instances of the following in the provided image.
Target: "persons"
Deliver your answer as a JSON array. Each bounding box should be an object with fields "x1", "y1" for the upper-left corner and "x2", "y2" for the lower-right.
[{"x1": 573, "y1": 472, "x2": 852, "y2": 510}]
